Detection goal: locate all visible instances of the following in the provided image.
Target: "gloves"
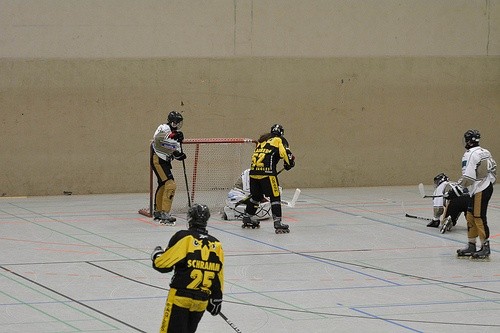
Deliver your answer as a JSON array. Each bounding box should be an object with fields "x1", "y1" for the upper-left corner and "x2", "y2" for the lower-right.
[
  {"x1": 151, "y1": 245, "x2": 164, "y2": 260},
  {"x1": 426, "y1": 219, "x2": 441, "y2": 228},
  {"x1": 284, "y1": 161, "x2": 295, "y2": 171},
  {"x1": 207, "y1": 299, "x2": 221, "y2": 316},
  {"x1": 444, "y1": 186, "x2": 464, "y2": 201},
  {"x1": 174, "y1": 131, "x2": 184, "y2": 142},
  {"x1": 173, "y1": 150, "x2": 186, "y2": 160}
]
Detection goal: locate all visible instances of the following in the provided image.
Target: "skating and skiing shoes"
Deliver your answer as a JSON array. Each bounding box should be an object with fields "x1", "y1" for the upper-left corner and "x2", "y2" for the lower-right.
[
  {"x1": 154, "y1": 211, "x2": 177, "y2": 226},
  {"x1": 242, "y1": 215, "x2": 260, "y2": 229},
  {"x1": 274, "y1": 220, "x2": 290, "y2": 233}
]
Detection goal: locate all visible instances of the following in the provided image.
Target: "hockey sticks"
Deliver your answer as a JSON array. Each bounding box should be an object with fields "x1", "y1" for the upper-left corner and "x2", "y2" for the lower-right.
[
  {"x1": 400, "y1": 201, "x2": 434, "y2": 221},
  {"x1": 218, "y1": 311, "x2": 242, "y2": 333},
  {"x1": 281, "y1": 188, "x2": 301, "y2": 208},
  {"x1": 418, "y1": 182, "x2": 469, "y2": 199},
  {"x1": 180, "y1": 142, "x2": 193, "y2": 209},
  {"x1": 277, "y1": 167, "x2": 285, "y2": 176}
]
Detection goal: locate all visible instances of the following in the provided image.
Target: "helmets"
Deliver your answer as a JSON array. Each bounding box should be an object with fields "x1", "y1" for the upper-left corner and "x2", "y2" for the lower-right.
[
  {"x1": 186, "y1": 203, "x2": 211, "y2": 229},
  {"x1": 433, "y1": 173, "x2": 448, "y2": 186},
  {"x1": 464, "y1": 129, "x2": 480, "y2": 148},
  {"x1": 271, "y1": 124, "x2": 285, "y2": 136},
  {"x1": 168, "y1": 111, "x2": 184, "y2": 131}
]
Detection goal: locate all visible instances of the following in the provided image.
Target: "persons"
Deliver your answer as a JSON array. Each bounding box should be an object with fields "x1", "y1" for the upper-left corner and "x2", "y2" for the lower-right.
[
  {"x1": 242, "y1": 124, "x2": 295, "y2": 229},
  {"x1": 427, "y1": 173, "x2": 470, "y2": 230},
  {"x1": 225, "y1": 168, "x2": 272, "y2": 221},
  {"x1": 151, "y1": 204, "x2": 224, "y2": 333},
  {"x1": 444, "y1": 129, "x2": 497, "y2": 257},
  {"x1": 150, "y1": 110, "x2": 186, "y2": 222}
]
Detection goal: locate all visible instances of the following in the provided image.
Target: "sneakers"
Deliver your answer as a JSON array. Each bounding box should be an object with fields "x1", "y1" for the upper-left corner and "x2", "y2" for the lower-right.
[
  {"x1": 439, "y1": 215, "x2": 453, "y2": 234},
  {"x1": 457, "y1": 241, "x2": 477, "y2": 259},
  {"x1": 470, "y1": 241, "x2": 491, "y2": 262}
]
